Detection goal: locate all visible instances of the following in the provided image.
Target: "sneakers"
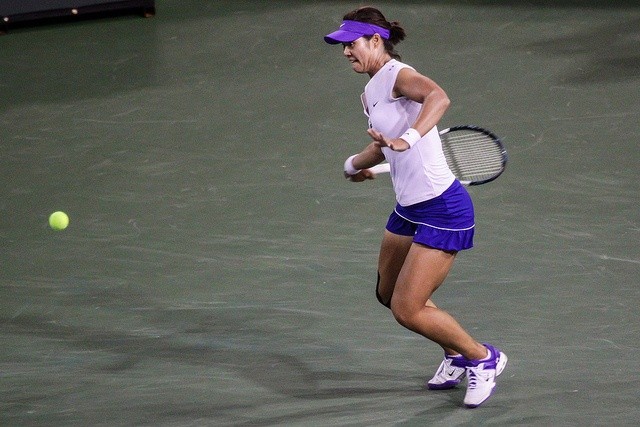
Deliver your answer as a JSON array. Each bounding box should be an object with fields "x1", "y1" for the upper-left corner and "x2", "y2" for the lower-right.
[
  {"x1": 464, "y1": 345, "x2": 507, "y2": 408},
  {"x1": 428, "y1": 352, "x2": 466, "y2": 389}
]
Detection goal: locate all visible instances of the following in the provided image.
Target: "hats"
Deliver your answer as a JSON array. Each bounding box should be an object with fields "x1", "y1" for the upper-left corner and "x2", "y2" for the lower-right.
[{"x1": 324, "y1": 21, "x2": 389, "y2": 45}]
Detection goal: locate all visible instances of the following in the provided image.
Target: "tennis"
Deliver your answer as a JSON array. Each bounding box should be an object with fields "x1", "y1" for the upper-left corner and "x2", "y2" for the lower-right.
[{"x1": 49, "y1": 211, "x2": 69, "y2": 232}]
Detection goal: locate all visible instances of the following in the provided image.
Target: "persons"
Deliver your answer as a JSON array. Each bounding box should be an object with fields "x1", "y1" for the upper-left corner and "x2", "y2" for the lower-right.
[{"x1": 323, "y1": 8, "x2": 507, "y2": 409}]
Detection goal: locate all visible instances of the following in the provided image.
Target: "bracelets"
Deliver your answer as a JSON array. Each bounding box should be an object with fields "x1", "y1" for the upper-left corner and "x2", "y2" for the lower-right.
[
  {"x1": 343, "y1": 153, "x2": 361, "y2": 177},
  {"x1": 398, "y1": 128, "x2": 422, "y2": 149}
]
